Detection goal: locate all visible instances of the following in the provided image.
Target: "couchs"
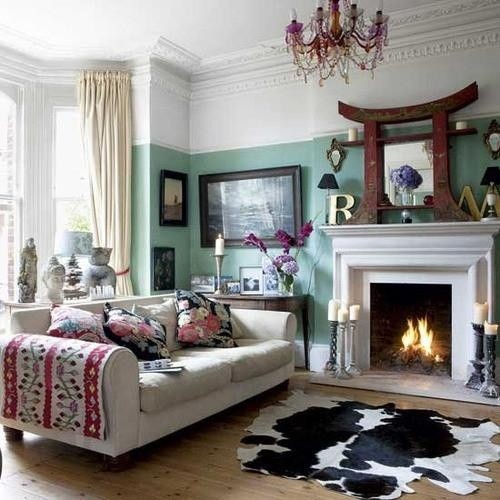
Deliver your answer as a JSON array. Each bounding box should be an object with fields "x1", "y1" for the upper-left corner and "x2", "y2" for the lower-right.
[{"x1": 0, "y1": 292, "x2": 301, "y2": 474}]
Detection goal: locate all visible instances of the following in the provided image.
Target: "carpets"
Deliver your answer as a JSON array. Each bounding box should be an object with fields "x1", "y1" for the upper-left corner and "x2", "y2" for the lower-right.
[{"x1": 240, "y1": 385, "x2": 500, "y2": 499}]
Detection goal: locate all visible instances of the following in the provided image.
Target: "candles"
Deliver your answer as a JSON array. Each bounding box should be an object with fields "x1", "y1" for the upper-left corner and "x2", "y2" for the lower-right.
[
  {"x1": 348, "y1": 126, "x2": 357, "y2": 142},
  {"x1": 454, "y1": 121, "x2": 468, "y2": 129},
  {"x1": 473, "y1": 301, "x2": 499, "y2": 335},
  {"x1": 327, "y1": 299, "x2": 362, "y2": 324},
  {"x1": 486, "y1": 194, "x2": 496, "y2": 206},
  {"x1": 215, "y1": 237, "x2": 225, "y2": 254}
]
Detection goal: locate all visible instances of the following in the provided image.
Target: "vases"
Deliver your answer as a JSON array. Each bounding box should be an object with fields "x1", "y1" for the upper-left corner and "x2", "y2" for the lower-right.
[
  {"x1": 277, "y1": 273, "x2": 295, "y2": 297},
  {"x1": 401, "y1": 190, "x2": 414, "y2": 206}
]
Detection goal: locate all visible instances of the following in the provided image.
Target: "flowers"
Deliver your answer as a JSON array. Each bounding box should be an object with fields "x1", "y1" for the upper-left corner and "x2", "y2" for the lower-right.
[
  {"x1": 389, "y1": 165, "x2": 423, "y2": 192},
  {"x1": 240, "y1": 210, "x2": 324, "y2": 294}
]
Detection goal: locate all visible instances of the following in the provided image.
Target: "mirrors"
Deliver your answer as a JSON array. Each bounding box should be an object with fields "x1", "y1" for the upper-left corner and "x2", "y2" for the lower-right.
[{"x1": 336, "y1": 80, "x2": 479, "y2": 224}]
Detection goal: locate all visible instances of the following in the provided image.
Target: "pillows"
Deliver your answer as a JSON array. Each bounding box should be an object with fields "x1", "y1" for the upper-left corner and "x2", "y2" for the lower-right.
[
  {"x1": 43, "y1": 301, "x2": 171, "y2": 361},
  {"x1": 174, "y1": 287, "x2": 239, "y2": 348}
]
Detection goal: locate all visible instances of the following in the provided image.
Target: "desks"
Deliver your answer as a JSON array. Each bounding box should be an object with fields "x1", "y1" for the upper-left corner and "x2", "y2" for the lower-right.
[{"x1": 209, "y1": 292, "x2": 312, "y2": 371}]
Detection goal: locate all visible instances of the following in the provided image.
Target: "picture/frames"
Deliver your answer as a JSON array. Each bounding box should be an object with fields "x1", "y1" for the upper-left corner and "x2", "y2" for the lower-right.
[
  {"x1": 152, "y1": 246, "x2": 176, "y2": 291},
  {"x1": 238, "y1": 266, "x2": 264, "y2": 296},
  {"x1": 159, "y1": 168, "x2": 189, "y2": 228},
  {"x1": 190, "y1": 272, "x2": 215, "y2": 295},
  {"x1": 224, "y1": 278, "x2": 240, "y2": 295},
  {"x1": 197, "y1": 164, "x2": 304, "y2": 249}
]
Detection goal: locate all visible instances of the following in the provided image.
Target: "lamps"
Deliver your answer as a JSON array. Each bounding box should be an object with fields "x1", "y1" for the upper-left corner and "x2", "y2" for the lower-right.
[
  {"x1": 284, "y1": 0, "x2": 391, "y2": 89},
  {"x1": 317, "y1": 173, "x2": 339, "y2": 225},
  {"x1": 56, "y1": 229, "x2": 94, "y2": 301},
  {"x1": 480, "y1": 165, "x2": 500, "y2": 193}
]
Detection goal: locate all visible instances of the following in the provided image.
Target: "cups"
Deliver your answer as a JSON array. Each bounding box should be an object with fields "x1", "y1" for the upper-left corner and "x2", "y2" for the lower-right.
[
  {"x1": 348, "y1": 128, "x2": 357, "y2": 141},
  {"x1": 455, "y1": 122, "x2": 467, "y2": 130}
]
